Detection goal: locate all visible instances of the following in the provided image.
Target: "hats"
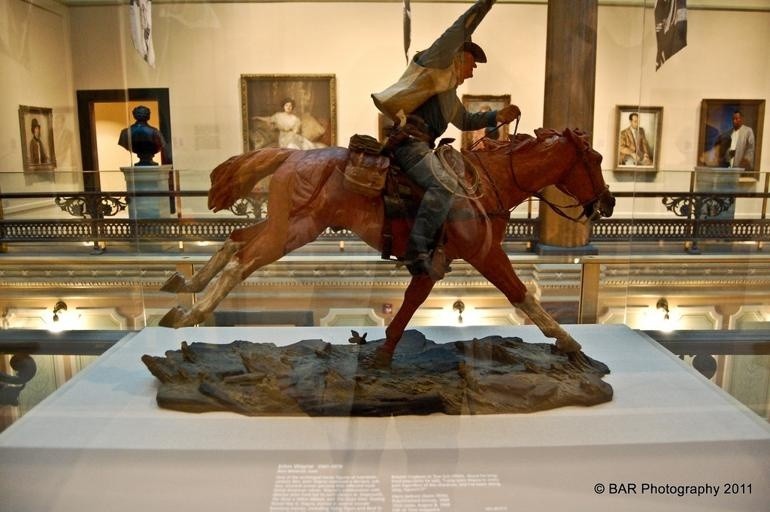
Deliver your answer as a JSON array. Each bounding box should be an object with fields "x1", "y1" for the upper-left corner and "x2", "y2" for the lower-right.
[{"x1": 31, "y1": 118, "x2": 41, "y2": 127}]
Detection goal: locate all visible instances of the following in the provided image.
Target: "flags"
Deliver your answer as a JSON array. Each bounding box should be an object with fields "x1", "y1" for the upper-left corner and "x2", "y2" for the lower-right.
[
  {"x1": 400, "y1": 1, "x2": 413, "y2": 65},
  {"x1": 128, "y1": 0, "x2": 157, "y2": 69},
  {"x1": 651, "y1": 0, "x2": 687, "y2": 72}
]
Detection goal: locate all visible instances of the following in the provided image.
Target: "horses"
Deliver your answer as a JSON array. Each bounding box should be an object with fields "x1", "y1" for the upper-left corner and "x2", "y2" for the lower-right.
[{"x1": 158, "y1": 127, "x2": 615, "y2": 368}]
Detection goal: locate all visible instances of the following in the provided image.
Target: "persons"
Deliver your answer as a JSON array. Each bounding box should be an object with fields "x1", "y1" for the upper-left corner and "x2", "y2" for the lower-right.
[
  {"x1": 619, "y1": 113, "x2": 652, "y2": 168},
  {"x1": 372, "y1": 0, "x2": 521, "y2": 278},
  {"x1": 251, "y1": 97, "x2": 317, "y2": 152},
  {"x1": 118, "y1": 106, "x2": 169, "y2": 166},
  {"x1": 713, "y1": 111, "x2": 756, "y2": 173},
  {"x1": 473, "y1": 105, "x2": 503, "y2": 150},
  {"x1": 29, "y1": 119, "x2": 50, "y2": 168}
]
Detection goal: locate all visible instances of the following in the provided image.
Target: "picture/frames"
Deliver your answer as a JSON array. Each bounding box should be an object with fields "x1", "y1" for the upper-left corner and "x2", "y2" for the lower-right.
[
  {"x1": 18, "y1": 102, "x2": 57, "y2": 174},
  {"x1": 240, "y1": 71, "x2": 338, "y2": 154},
  {"x1": 614, "y1": 104, "x2": 664, "y2": 173},
  {"x1": 461, "y1": 93, "x2": 511, "y2": 148},
  {"x1": 697, "y1": 99, "x2": 766, "y2": 182}
]
